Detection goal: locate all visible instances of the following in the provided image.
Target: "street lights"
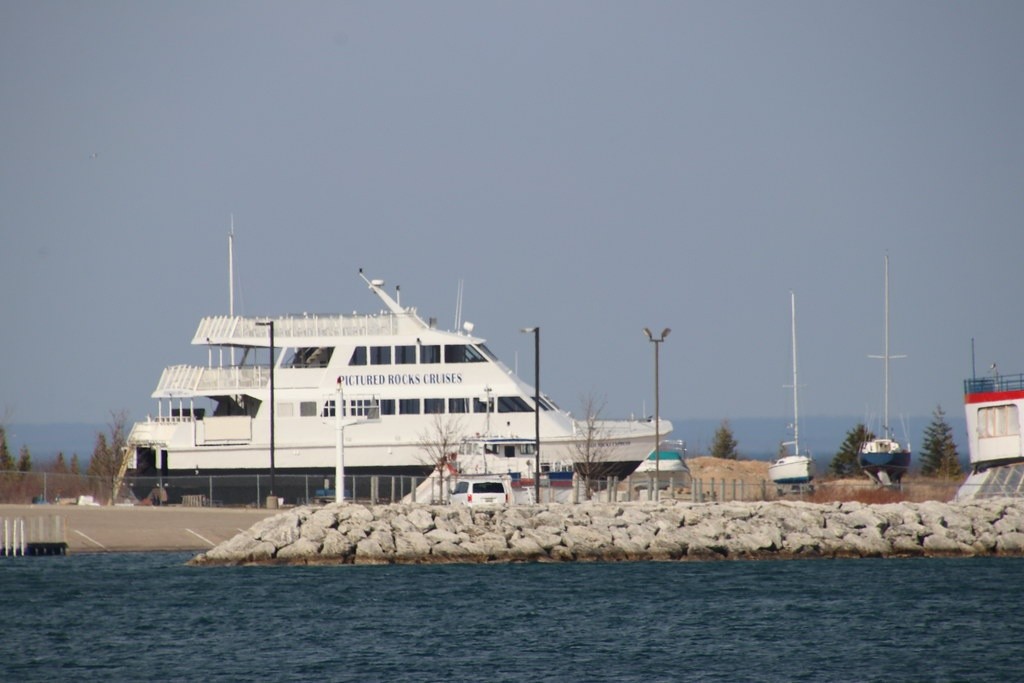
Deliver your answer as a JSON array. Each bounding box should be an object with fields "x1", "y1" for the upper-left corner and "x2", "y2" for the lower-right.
[
  {"x1": 521, "y1": 326, "x2": 539, "y2": 503},
  {"x1": 642, "y1": 327, "x2": 672, "y2": 501},
  {"x1": 255, "y1": 322, "x2": 275, "y2": 495}
]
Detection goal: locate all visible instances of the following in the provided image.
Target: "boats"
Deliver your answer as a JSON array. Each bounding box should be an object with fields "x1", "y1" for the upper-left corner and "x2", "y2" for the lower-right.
[
  {"x1": 397, "y1": 388, "x2": 573, "y2": 505},
  {"x1": 113, "y1": 232, "x2": 673, "y2": 505},
  {"x1": 628, "y1": 440, "x2": 692, "y2": 500}
]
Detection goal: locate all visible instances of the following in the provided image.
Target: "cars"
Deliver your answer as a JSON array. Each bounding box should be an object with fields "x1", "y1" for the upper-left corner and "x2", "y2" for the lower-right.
[{"x1": 449, "y1": 477, "x2": 508, "y2": 507}]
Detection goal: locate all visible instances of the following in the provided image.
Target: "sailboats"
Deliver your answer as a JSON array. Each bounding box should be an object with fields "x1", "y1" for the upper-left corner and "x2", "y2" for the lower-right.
[
  {"x1": 858, "y1": 254, "x2": 912, "y2": 490},
  {"x1": 768, "y1": 290, "x2": 817, "y2": 483}
]
